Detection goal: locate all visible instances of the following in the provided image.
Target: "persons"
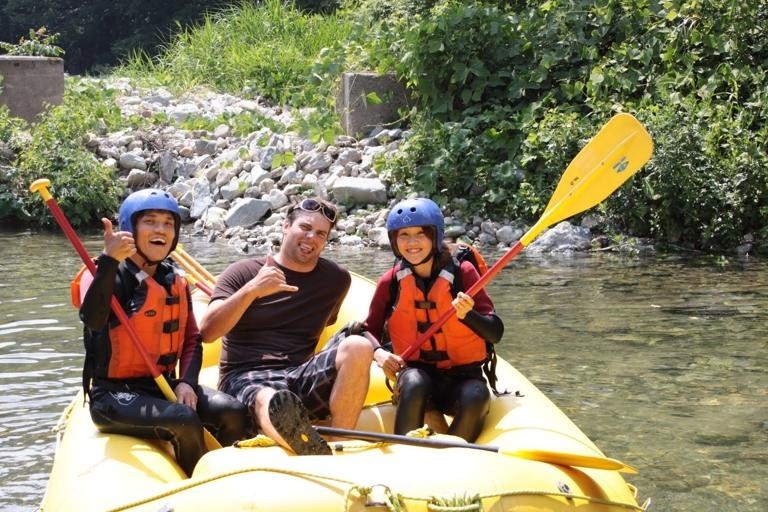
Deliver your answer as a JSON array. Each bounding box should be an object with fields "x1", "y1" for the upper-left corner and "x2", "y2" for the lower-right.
[
  {"x1": 363, "y1": 196, "x2": 507, "y2": 447},
  {"x1": 71, "y1": 188, "x2": 248, "y2": 480},
  {"x1": 196, "y1": 194, "x2": 376, "y2": 458}
]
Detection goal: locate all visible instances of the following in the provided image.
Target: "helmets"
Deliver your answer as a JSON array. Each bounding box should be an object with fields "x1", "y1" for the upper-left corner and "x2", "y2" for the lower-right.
[
  {"x1": 118, "y1": 188, "x2": 181, "y2": 250},
  {"x1": 387, "y1": 197, "x2": 445, "y2": 258}
]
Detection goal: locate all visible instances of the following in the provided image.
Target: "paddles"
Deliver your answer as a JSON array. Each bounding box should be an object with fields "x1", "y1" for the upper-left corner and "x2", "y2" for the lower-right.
[
  {"x1": 29, "y1": 178, "x2": 222, "y2": 451},
  {"x1": 383, "y1": 113, "x2": 653, "y2": 383},
  {"x1": 312, "y1": 426, "x2": 637, "y2": 473}
]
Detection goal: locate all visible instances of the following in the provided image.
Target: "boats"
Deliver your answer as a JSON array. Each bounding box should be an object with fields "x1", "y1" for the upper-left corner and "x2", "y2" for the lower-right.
[{"x1": 33, "y1": 267, "x2": 655, "y2": 512}]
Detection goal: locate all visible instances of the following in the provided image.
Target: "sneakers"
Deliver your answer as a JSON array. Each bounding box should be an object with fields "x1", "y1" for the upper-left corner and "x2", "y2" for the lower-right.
[{"x1": 268, "y1": 390, "x2": 333, "y2": 455}]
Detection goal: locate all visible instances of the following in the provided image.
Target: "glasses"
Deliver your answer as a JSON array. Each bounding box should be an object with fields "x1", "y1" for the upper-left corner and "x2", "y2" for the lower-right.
[{"x1": 288, "y1": 198, "x2": 338, "y2": 226}]
